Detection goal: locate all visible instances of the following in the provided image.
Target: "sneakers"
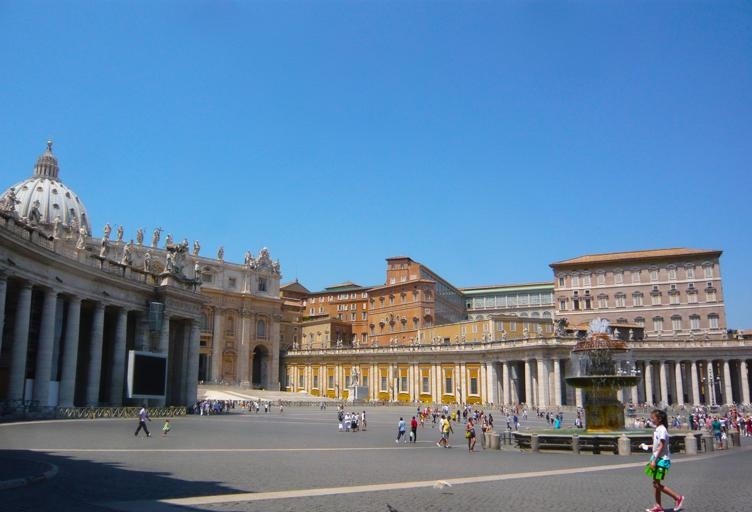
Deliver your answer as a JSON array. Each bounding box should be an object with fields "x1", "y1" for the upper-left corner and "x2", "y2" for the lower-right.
[
  {"x1": 646, "y1": 504, "x2": 664, "y2": 512},
  {"x1": 673, "y1": 495, "x2": 684, "y2": 512}
]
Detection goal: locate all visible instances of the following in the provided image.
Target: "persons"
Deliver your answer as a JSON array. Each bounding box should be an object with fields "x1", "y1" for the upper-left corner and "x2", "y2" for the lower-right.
[
  {"x1": 320, "y1": 394, "x2": 329, "y2": 412},
  {"x1": 134, "y1": 404, "x2": 151, "y2": 438},
  {"x1": 395, "y1": 401, "x2": 585, "y2": 454},
  {"x1": 625, "y1": 400, "x2": 752, "y2": 449},
  {"x1": 280, "y1": 404, "x2": 283, "y2": 415},
  {"x1": 193, "y1": 398, "x2": 271, "y2": 416},
  {"x1": 336, "y1": 402, "x2": 367, "y2": 432},
  {"x1": 639, "y1": 408, "x2": 684, "y2": 512},
  {"x1": 163, "y1": 418, "x2": 171, "y2": 434}
]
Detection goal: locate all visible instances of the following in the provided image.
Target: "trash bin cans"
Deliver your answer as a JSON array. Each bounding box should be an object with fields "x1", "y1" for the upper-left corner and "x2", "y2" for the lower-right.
[
  {"x1": 703, "y1": 437, "x2": 714, "y2": 452},
  {"x1": 730, "y1": 432, "x2": 740, "y2": 447}
]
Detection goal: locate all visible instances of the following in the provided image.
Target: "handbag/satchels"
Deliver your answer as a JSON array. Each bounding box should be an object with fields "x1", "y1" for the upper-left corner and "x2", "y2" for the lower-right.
[{"x1": 465, "y1": 431, "x2": 472, "y2": 438}]
